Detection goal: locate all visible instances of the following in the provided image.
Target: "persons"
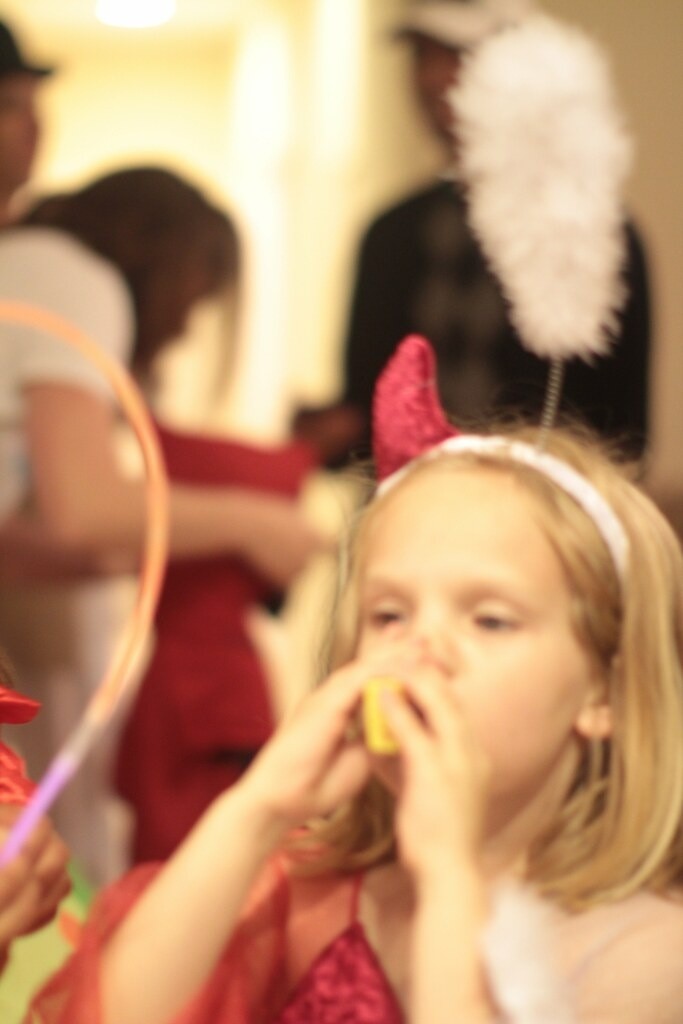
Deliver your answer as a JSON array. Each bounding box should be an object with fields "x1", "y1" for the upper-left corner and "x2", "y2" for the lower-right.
[
  {"x1": 19, "y1": 424, "x2": 683, "y2": 1022},
  {"x1": 0, "y1": 17, "x2": 319, "y2": 996},
  {"x1": 294, "y1": 0, "x2": 651, "y2": 465}
]
